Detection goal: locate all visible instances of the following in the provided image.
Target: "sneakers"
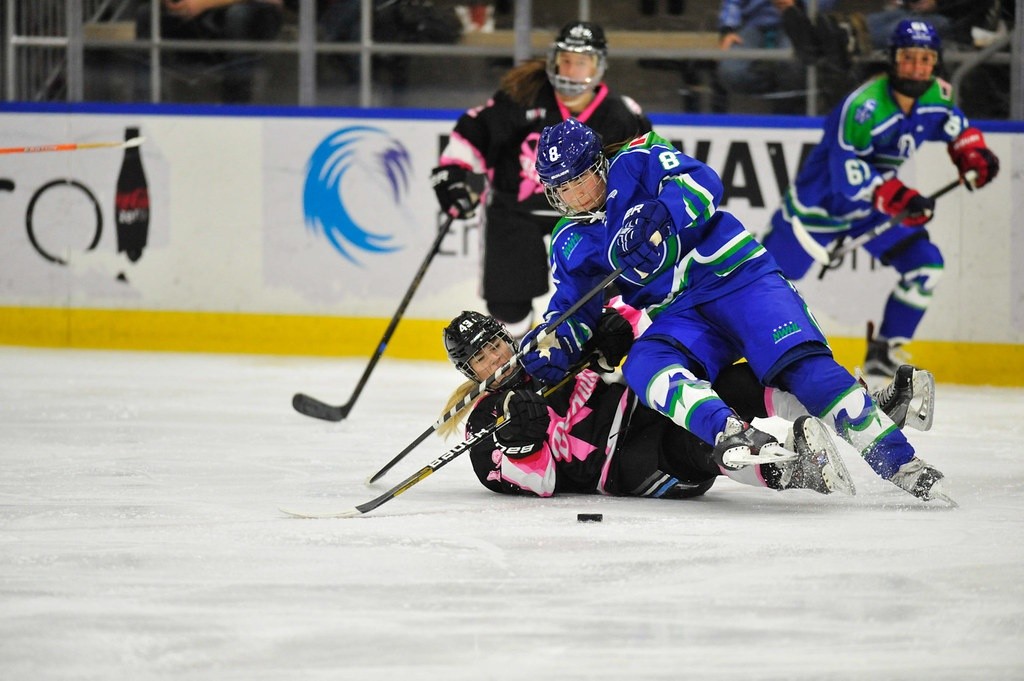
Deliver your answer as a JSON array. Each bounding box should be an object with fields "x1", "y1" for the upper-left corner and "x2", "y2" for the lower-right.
[
  {"x1": 759, "y1": 415, "x2": 855, "y2": 496},
  {"x1": 856, "y1": 321, "x2": 904, "y2": 393},
  {"x1": 886, "y1": 456, "x2": 960, "y2": 508},
  {"x1": 872, "y1": 364, "x2": 935, "y2": 431},
  {"x1": 711, "y1": 406, "x2": 803, "y2": 470}
]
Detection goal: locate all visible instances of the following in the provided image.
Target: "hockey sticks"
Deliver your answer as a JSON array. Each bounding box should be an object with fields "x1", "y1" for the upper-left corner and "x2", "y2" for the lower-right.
[
  {"x1": 788, "y1": 170, "x2": 974, "y2": 267},
  {"x1": 325, "y1": 264, "x2": 628, "y2": 491},
  {"x1": 286, "y1": 213, "x2": 458, "y2": 425},
  {"x1": 0, "y1": 135, "x2": 150, "y2": 155},
  {"x1": 273, "y1": 347, "x2": 604, "y2": 522}
]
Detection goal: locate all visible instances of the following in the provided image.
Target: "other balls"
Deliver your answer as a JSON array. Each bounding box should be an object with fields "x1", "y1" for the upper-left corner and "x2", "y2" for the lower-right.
[{"x1": 576, "y1": 513, "x2": 603, "y2": 522}]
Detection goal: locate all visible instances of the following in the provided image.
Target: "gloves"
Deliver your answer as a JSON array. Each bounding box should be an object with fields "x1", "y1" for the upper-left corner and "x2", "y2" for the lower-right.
[
  {"x1": 580, "y1": 309, "x2": 637, "y2": 374},
  {"x1": 871, "y1": 176, "x2": 936, "y2": 226},
  {"x1": 515, "y1": 321, "x2": 570, "y2": 382},
  {"x1": 491, "y1": 390, "x2": 551, "y2": 458},
  {"x1": 614, "y1": 198, "x2": 674, "y2": 283},
  {"x1": 429, "y1": 165, "x2": 487, "y2": 220},
  {"x1": 949, "y1": 128, "x2": 1001, "y2": 194}
]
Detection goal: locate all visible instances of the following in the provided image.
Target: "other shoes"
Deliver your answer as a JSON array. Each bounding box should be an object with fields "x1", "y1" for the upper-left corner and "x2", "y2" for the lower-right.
[
  {"x1": 816, "y1": 10, "x2": 850, "y2": 57},
  {"x1": 781, "y1": 6, "x2": 819, "y2": 64}
]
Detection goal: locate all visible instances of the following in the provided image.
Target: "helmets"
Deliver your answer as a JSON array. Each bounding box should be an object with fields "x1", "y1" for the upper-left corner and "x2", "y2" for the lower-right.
[
  {"x1": 886, "y1": 18, "x2": 941, "y2": 98},
  {"x1": 536, "y1": 117, "x2": 611, "y2": 222},
  {"x1": 444, "y1": 313, "x2": 526, "y2": 392},
  {"x1": 544, "y1": 22, "x2": 610, "y2": 96}
]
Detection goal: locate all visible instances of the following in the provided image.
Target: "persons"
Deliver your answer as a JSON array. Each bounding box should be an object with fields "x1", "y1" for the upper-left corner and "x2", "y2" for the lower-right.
[
  {"x1": 519, "y1": 119, "x2": 960, "y2": 509},
  {"x1": 717, "y1": 0, "x2": 1015, "y2": 115},
  {"x1": 763, "y1": 20, "x2": 999, "y2": 392},
  {"x1": 443, "y1": 311, "x2": 869, "y2": 500},
  {"x1": 433, "y1": 22, "x2": 644, "y2": 347},
  {"x1": 128, "y1": 2, "x2": 465, "y2": 106}
]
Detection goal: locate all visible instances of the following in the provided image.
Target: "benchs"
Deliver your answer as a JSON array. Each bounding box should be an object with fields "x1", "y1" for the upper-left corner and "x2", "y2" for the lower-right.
[{"x1": 81, "y1": 21, "x2": 727, "y2": 117}]
